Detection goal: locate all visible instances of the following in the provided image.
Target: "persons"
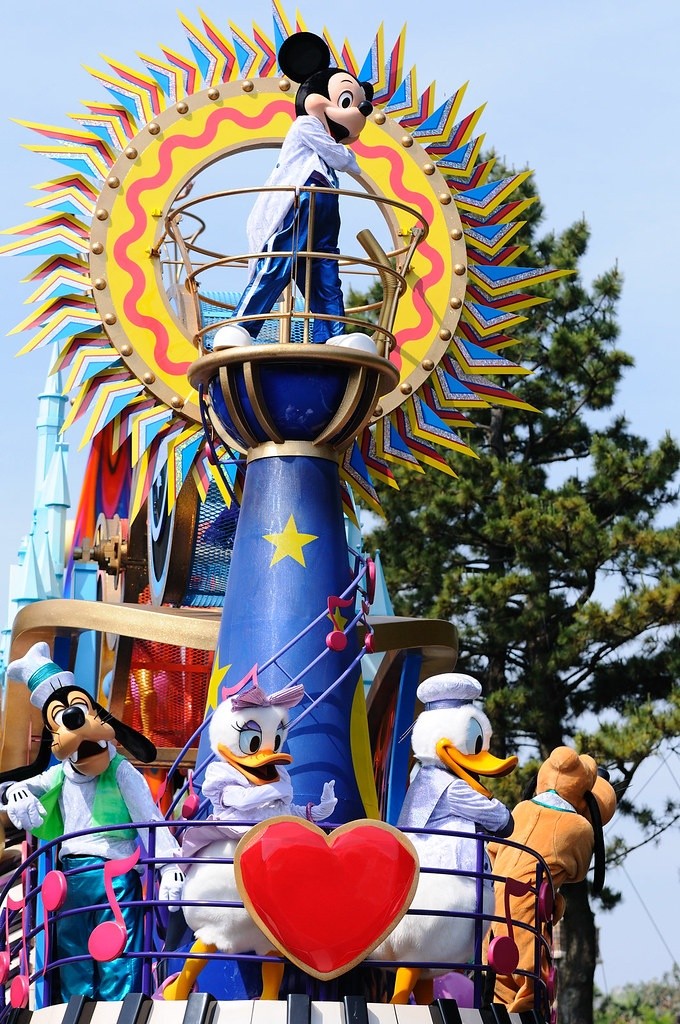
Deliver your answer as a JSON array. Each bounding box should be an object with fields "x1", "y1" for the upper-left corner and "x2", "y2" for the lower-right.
[{"x1": 212, "y1": 32, "x2": 381, "y2": 353}]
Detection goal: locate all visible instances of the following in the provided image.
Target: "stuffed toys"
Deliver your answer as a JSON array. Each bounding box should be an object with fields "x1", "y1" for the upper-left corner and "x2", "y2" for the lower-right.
[
  {"x1": 489, "y1": 744, "x2": 616, "y2": 1013},
  {"x1": 368, "y1": 672, "x2": 514, "y2": 1006},
  {"x1": 151, "y1": 684, "x2": 336, "y2": 1001},
  {"x1": 1, "y1": 682, "x2": 184, "y2": 1009}
]
